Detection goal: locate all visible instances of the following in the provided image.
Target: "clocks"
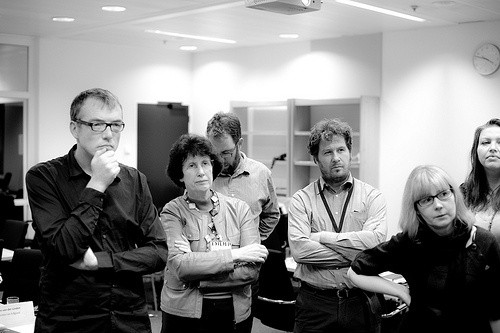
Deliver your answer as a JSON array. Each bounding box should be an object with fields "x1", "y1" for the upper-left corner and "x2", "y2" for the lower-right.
[{"x1": 472, "y1": 43, "x2": 500, "y2": 76}]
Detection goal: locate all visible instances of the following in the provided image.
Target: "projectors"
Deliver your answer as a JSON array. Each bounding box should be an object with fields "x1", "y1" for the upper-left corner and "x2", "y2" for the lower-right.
[{"x1": 245, "y1": 0, "x2": 321, "y2": 15}]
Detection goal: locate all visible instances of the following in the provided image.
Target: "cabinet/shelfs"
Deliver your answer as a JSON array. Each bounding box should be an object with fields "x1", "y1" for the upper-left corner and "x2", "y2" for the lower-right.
[
  {"x1": 290, "y1": 95, "x2": 380, "y2": 197},
  {"x1": 230, "y1": 99, "x2": 290, "y2": 197}
]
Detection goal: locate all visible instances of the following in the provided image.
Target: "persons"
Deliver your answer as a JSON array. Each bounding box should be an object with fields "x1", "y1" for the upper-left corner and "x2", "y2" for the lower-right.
[
  {"x1": 206, "y1": 111, "x2": 280, "y2": 333},
  {"x1": 159, "y1": 133, "x2": 269, "y2": 333},
  {"x1": 288, "y1": 120, "x2": 388, "y2": 333},
  {"x1": 25, "y1": 88, "x2": 168, "y2": 333},
  {"x1": 455, "y1": 118, "x2": 500, "y2": 240},
  {"x1": 346, "y1": 164, "x2": 500, "y2": 333}
]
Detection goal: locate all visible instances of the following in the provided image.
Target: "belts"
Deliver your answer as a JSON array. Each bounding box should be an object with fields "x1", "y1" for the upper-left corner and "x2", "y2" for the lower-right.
[{"x1": 301, "y1": 282, "x2": 362, "y2": 299}]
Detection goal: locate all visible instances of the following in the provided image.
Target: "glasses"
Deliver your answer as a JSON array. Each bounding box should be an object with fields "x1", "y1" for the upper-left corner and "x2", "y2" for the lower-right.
[
  {"x1": 414, "y1": 188, "x2": 454, "y2": 206},
  {"x1": 73, "y1": 118, "x2": 126, "y2": 133},
  {"x1": 222, "y1": 145, "x2": 236, "y2": 158}
]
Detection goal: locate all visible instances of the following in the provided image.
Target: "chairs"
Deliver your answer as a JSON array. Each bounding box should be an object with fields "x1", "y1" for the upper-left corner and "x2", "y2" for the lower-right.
[{"x1": 0, "y1": 219, "x2": 43, "y2": 306}]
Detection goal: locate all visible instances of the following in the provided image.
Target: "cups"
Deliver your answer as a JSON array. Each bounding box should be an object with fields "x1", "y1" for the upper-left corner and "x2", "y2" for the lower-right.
[{"x1": 7, "y1": 297, "x2": 19, "y2": 304}]
